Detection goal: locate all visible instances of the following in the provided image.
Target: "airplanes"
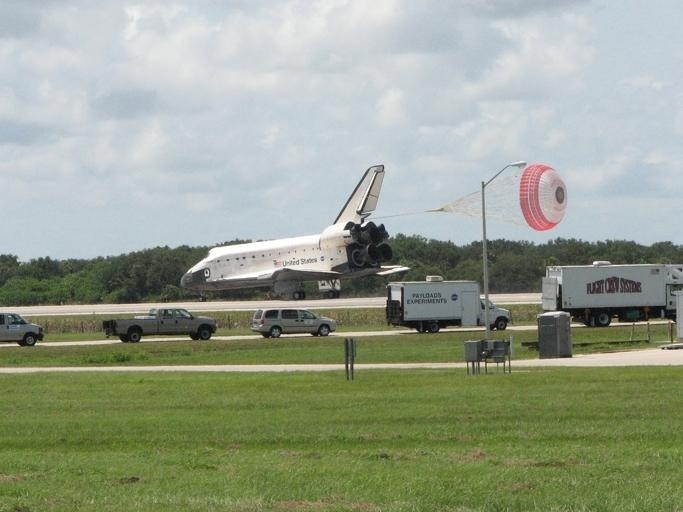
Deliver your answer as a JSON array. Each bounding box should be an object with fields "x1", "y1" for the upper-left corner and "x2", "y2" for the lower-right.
[{"x1": 179, "y1": 164, "x2": 411, "y2": 302}]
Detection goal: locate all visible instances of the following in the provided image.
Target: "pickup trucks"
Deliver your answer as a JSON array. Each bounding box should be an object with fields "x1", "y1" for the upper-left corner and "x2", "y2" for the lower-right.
[{"x1": 102, "y1": 307, "x2": 217, "y2": 343}]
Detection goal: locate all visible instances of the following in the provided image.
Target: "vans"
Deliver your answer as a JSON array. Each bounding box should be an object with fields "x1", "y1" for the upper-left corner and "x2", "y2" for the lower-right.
[
  {"x1": 251, "y1": 307, "x2": 337, "y2": 338},
  {"x1": 0, "y1": 313, "x2": 44, "y2": 347}
]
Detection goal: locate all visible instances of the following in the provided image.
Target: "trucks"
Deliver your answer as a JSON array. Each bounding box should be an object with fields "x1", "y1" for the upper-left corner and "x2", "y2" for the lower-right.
[
  {"x1": 386, "y1": 275, "x2": 511, "y2": 334},
  {"x1": 542, "y1": 260, "x2": 683, "y2": 327}
]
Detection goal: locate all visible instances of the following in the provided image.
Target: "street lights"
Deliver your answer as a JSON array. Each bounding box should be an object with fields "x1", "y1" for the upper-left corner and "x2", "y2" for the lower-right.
[{"x1": 482, "y1": 161, "x2": 527, "y2": 340}]
